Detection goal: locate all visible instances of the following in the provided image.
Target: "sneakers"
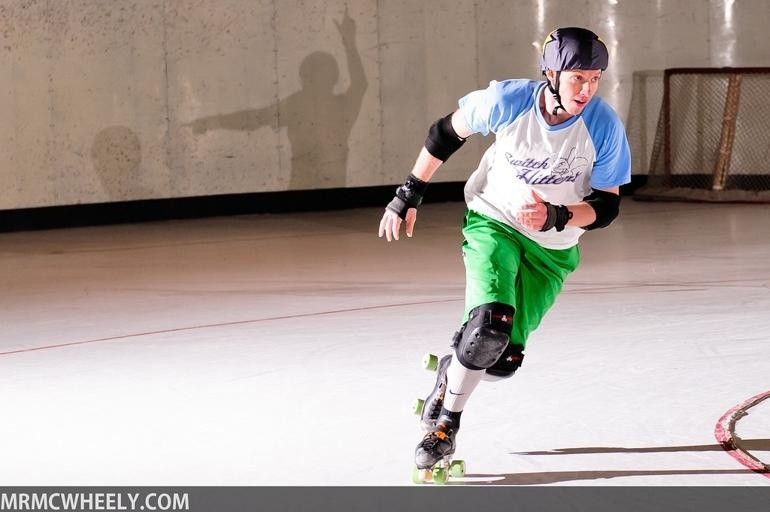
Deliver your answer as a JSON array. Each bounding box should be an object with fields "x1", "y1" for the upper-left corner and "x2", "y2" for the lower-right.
[{"x1": 414, "y1": 353, "x2": 457, "y2": 470}]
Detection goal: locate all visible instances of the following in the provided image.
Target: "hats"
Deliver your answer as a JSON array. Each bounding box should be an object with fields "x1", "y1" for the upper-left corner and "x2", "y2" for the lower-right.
[{"x1": 540, "y1": 26, "x2": 609, "y2": 72}]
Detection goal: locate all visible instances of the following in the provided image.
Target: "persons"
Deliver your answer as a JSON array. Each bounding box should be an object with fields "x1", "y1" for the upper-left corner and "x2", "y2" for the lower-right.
[{"x1": 378, "y1": 26, "x2": 635, "y2": 473}]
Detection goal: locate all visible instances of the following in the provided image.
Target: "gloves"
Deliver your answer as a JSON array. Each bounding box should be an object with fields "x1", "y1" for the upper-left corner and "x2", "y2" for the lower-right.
[
  {"x1": 385, "y1": 173, "x2": 429, "y2": 221},
  {"x1": 538, "y1": 201, "x2": 573, "y2": 233}
]
